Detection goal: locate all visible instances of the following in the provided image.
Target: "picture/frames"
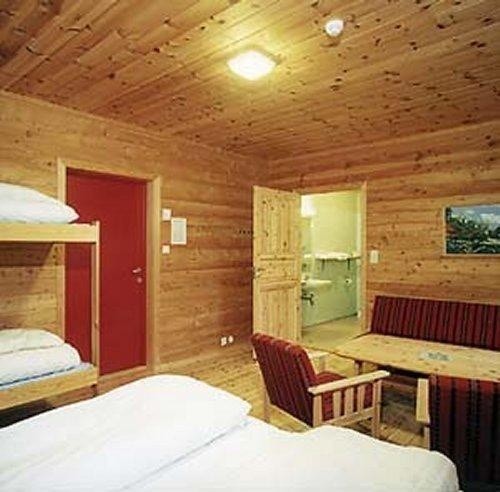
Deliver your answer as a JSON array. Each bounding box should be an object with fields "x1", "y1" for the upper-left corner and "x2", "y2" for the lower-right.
[{"x1": 443, "y1": 204, "x2": 500, "y2": 259}]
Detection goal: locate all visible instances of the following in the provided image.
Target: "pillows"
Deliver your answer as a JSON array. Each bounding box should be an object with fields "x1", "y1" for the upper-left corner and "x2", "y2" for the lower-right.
[{"x1": 0, "y1": 181, "x2": 78, "y2": 222}]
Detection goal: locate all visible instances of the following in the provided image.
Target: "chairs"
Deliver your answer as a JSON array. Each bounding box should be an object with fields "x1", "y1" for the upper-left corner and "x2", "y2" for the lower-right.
[
  {"x1": 250, "y1": 333, "x2": 391, "y2": 439},
  {"x1": 412, "y1": 377, "x2": 498, "y2": 492}
]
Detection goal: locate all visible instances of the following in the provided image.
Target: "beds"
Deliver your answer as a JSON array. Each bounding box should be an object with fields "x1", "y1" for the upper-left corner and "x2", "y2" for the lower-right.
[
  {"x1": 0, "y1": 372, "x2": 455, "y2": 492},
  {"x1": 0, "y1": 220, "x2": 102, "y2": 421}
]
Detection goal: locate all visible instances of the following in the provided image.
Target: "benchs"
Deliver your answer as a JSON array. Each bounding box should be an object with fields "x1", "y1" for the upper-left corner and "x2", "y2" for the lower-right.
[{"x1": 353, "y1": 293, "x2": 500, "y2": 355}]
